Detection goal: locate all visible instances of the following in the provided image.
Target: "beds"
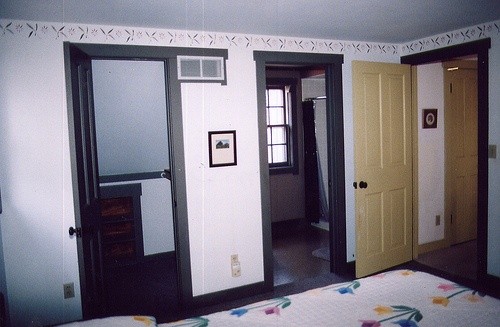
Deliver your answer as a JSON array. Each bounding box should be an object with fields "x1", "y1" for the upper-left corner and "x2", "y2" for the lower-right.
[{"x1": 56, "y1": 268, "x2": 500, "y2": 327}]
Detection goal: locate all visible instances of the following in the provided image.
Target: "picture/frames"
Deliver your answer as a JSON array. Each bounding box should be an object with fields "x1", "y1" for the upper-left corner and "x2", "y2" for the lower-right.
[
  {"x1": 208, "y1": 130, "x2": 237, "y2": 167},
  {"x1": 422, "y1": 108, "x2": 437, "y2": 129}
]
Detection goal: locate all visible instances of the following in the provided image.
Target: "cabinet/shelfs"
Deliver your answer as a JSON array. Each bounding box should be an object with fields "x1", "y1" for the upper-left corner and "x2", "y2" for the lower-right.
[{"x1": 98, "y1": 183, "x2": 145, "y2": 260}]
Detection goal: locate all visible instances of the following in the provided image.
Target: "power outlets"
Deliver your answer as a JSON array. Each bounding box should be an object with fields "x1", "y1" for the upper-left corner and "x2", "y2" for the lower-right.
[
  {"x1": 63, "y1": 283, "x2": 76, "y2": 299},
  {"x1": 231, "y1": 255, "x2": 241, "y2": 277}
]
